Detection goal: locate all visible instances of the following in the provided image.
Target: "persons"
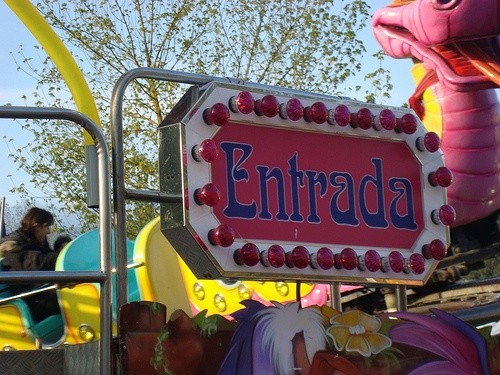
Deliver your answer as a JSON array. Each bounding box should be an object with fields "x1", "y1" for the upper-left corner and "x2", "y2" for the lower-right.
[{"x1": 0, "y1": 207, "x2": 63, "y2": 315}]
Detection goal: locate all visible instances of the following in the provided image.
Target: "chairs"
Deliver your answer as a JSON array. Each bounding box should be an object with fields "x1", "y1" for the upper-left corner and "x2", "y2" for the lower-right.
[
  {"x1": 0, "y1": 257, "x2": 63, "y2": 351},
  {"x1": 55, "y1": 229, "x2": 135, "y2": 344},
  {"x1": 133, "y1": 217, "x2": 193, "y2": 323}
]
